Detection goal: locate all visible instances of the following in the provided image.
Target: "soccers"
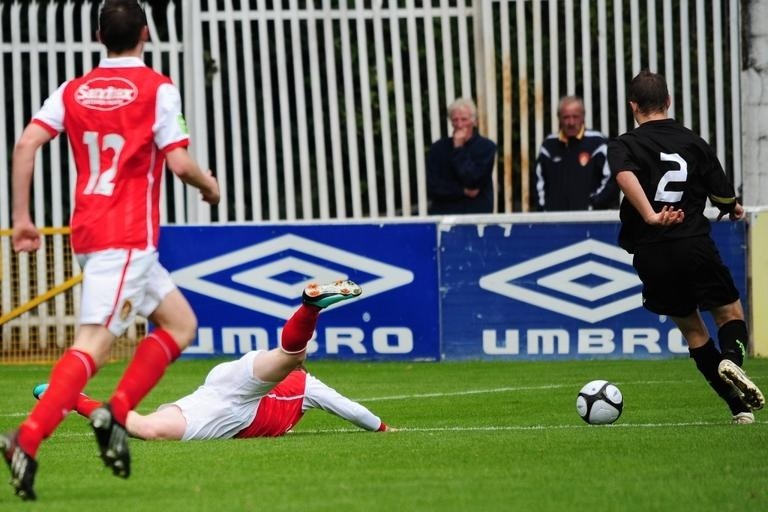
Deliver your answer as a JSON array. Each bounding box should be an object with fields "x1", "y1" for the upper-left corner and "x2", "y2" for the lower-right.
[{"x1": 576, "y1": 380, "x2": 623, "y2": 425}]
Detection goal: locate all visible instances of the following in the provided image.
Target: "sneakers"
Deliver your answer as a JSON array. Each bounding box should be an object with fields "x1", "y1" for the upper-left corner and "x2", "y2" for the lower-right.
[
  {"x1": 0, "y1": 430, "x2": 37, "y2": 500},
  {"x1": 88, "y1": 402, "x2": 129, "y2": 479},
  {"x1": 32, "y1": 383, "x2": 88, "y2": 411},
  {"x1": 302, "y1": 280, "x2": 361, "y2": 308},
  {"x1": 716, "y1": 359, "x2": 764, "y2": 411},
  {"x1": 731, "y1": 413, "x2": 754, "y2": 425}
]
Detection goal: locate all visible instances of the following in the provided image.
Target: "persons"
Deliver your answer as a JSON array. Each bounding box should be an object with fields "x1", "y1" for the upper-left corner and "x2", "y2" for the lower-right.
[
  {"x1": 426, "y1": 97, "x2": 496, "y2": 216},
  {"x1": 0, "y1": 0, "x2": 220, "y2": 501},
  {"x1": 32, "y1": 278, "x2": 396, "y2": 442},
  {"x1": 607, "y1": 72, "x2": 766, "y2": 426},
  {"x1": 534, "y1": 96, "x2": 620, "y2": 213}
]
